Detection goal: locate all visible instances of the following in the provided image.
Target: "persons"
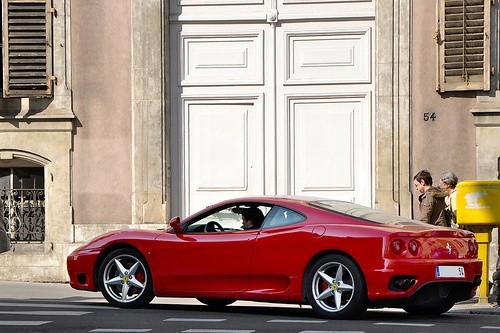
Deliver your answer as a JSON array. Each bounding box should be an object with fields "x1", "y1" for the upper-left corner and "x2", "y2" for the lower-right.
[
  {"x1": 439, "y1": 171, "x2": 470, "y2": 230},
  {"x1": 413, "y1": 169, "x2": 447, "y2": 227},
  {"x1": 240, "y1": 208, "x2": 265, "y2": 230}
]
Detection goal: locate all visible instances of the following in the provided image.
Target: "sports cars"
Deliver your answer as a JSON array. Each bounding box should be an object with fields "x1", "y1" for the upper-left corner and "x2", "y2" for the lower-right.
[{"x1": 67, "y1": 196, "x2": 484, "y2": 321}]
